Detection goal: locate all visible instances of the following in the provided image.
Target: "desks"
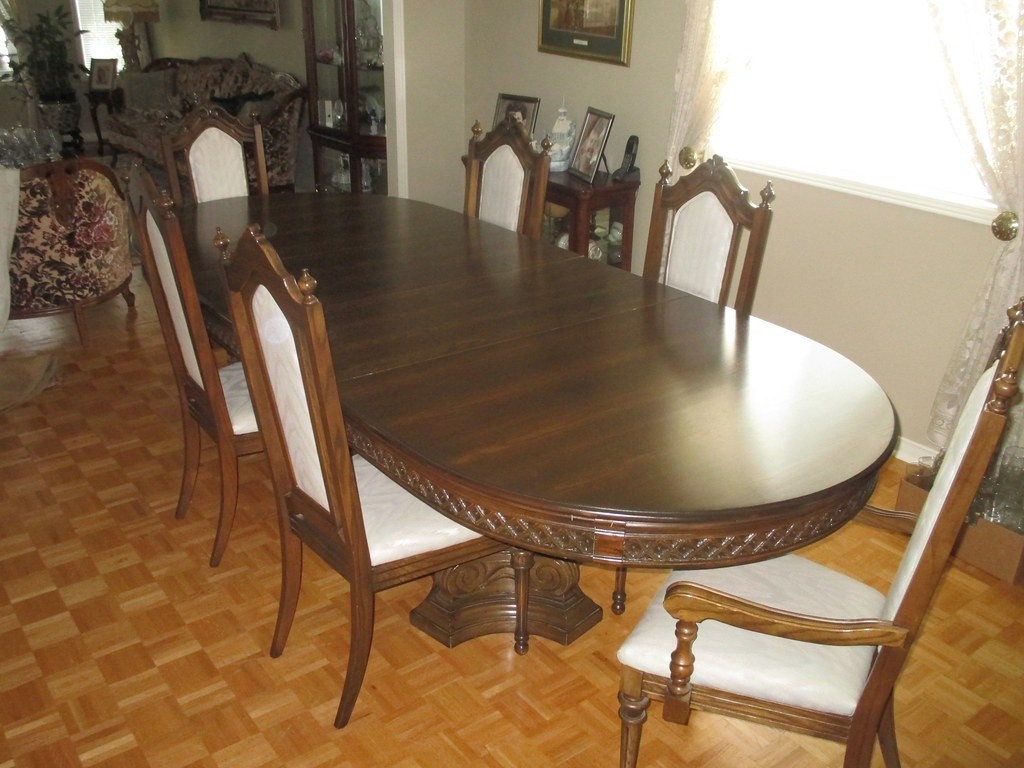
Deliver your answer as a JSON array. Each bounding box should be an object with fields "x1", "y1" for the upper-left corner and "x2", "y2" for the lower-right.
[{"x1": 174, "y1": 191, "x2": 901, "y2": 649}]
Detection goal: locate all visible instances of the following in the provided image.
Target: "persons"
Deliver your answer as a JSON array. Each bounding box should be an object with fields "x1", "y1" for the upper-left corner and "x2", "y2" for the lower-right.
[
  {"x1": 574, "y1": 117, "x2": 605, "y2": 176},
  {"x1": 504, "y1": 101, "x2": 527, "y2": 125}
]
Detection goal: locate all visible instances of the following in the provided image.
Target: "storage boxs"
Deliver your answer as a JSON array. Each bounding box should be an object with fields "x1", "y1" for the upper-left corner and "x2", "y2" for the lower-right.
[{"x1": 894, "y1": 468, "x2": 1024, "y2": 584}]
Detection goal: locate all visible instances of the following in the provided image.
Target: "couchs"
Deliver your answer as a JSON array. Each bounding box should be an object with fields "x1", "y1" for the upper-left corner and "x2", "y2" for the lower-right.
[{"x1": 102, "y1": 51, "x2": 308, "y2": 200}]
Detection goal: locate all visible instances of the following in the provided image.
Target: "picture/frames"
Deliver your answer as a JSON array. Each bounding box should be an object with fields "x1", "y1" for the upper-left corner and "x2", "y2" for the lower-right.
[
  {"x1": 88, "y1": 59, "x2": 117, "y2": 91},
  {"x1": 566, "y1": 104, "x2": 615, "y2": 183},
  {"x1": 199, "y1": 0, "x2": 281, "y2": 32},
  {"x1": 491, "y1": 93, "x2": 542, "y2": 139},
  {"x1": 537, "y1": 0, "x2": 634, "y2": 68}
]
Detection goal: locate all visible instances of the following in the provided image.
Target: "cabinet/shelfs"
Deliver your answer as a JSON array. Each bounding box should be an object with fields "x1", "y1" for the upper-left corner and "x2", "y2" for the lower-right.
[
  {"x1": 300, "y1": 0, "x2": 387, "y2": 193},
  {"x1": 461, "y1": 154, "x2": 639, "y2": 272}
]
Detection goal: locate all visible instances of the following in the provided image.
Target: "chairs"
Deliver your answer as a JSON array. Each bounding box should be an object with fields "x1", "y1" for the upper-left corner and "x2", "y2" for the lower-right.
[
  {"x1": 615, "y1": 294, "x2": 1024, "y2": 768},
  {"x1": 211, "y1": 219, "x2": 533, "y2": 731},
  {"x1": 120, "y1": 157, "x2": 266, "y2": 570},
  {"x1": 158, "y1": 98, "x2": 268, "y2": 205},
  {"x1": 610, "y1": 152, "x2": 776, "y2": 616},
  {"x1": 460, "y1": 109, "x2": 553, "y2": 241},
  {"x1": 9, "y1": 158, "x2": 136, "y2": 348}
]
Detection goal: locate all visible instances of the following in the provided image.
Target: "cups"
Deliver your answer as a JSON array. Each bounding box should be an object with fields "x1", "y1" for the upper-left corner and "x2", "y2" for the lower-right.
[{"x1": 906, "y1": 446, "x2": 1024, "y2": 536}]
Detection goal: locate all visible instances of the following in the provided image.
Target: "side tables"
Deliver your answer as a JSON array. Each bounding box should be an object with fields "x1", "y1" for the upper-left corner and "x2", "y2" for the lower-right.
[{"x1": 83, "y1": 87, "x2": 127, "y2": 157}]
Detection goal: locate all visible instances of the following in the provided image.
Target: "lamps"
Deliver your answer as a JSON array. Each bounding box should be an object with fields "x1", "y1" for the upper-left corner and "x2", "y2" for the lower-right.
[{"x1": 102, "y1": 0, "x2": 161, "y2": 71}]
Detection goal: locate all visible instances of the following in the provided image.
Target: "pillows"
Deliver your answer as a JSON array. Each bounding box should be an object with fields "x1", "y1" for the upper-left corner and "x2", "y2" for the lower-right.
[{"x1": 117, "y1": 66, "x2": 180, "y2": 114}]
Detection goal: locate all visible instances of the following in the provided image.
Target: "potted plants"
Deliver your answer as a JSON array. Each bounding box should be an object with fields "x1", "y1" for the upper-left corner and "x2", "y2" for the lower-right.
[{"x1": 0, "y1": 4, "x2": 92, "y2": 154}]
